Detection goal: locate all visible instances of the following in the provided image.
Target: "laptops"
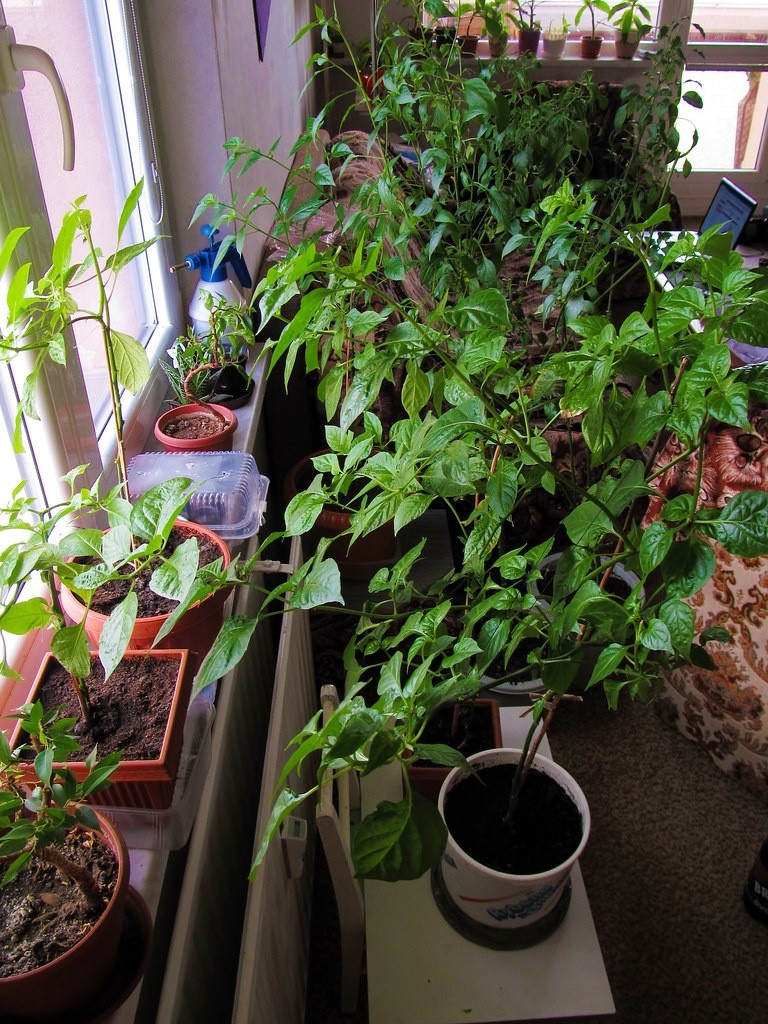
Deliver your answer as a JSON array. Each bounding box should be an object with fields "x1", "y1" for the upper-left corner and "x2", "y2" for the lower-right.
[{"x1": 642, "y1": 178, "x2": 757, "y2": 270}]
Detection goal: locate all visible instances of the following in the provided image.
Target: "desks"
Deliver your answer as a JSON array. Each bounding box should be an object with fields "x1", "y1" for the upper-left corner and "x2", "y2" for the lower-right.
[
  {"x1": 363, "y1": 705, "x2": 621, "y2": 1023},
  {"x1": 624, "y1": 230, "x2": 768, "y2": 368}
]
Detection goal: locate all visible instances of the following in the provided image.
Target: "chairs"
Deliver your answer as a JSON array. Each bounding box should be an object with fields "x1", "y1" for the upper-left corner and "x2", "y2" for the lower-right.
[{"x1": 315, "y1": 685, "x2": 366, "y2": 1014}]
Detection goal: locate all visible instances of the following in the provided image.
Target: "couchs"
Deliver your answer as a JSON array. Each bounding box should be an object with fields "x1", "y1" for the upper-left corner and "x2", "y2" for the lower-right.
[
  {"x1": 253, "y1": 129, "x2": 660, "y2": 543},
  {"x1": 476, "y1": 80, "x2": 683, "y2": 300},
  {"x1": 641, "y1": 403, "x2": 768, "y2": 803}
]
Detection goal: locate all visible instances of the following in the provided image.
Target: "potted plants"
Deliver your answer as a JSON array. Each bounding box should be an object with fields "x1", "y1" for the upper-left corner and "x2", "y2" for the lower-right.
[{"x1": 2, "y1": 1, "x2": 768, "y2": 1022}]
